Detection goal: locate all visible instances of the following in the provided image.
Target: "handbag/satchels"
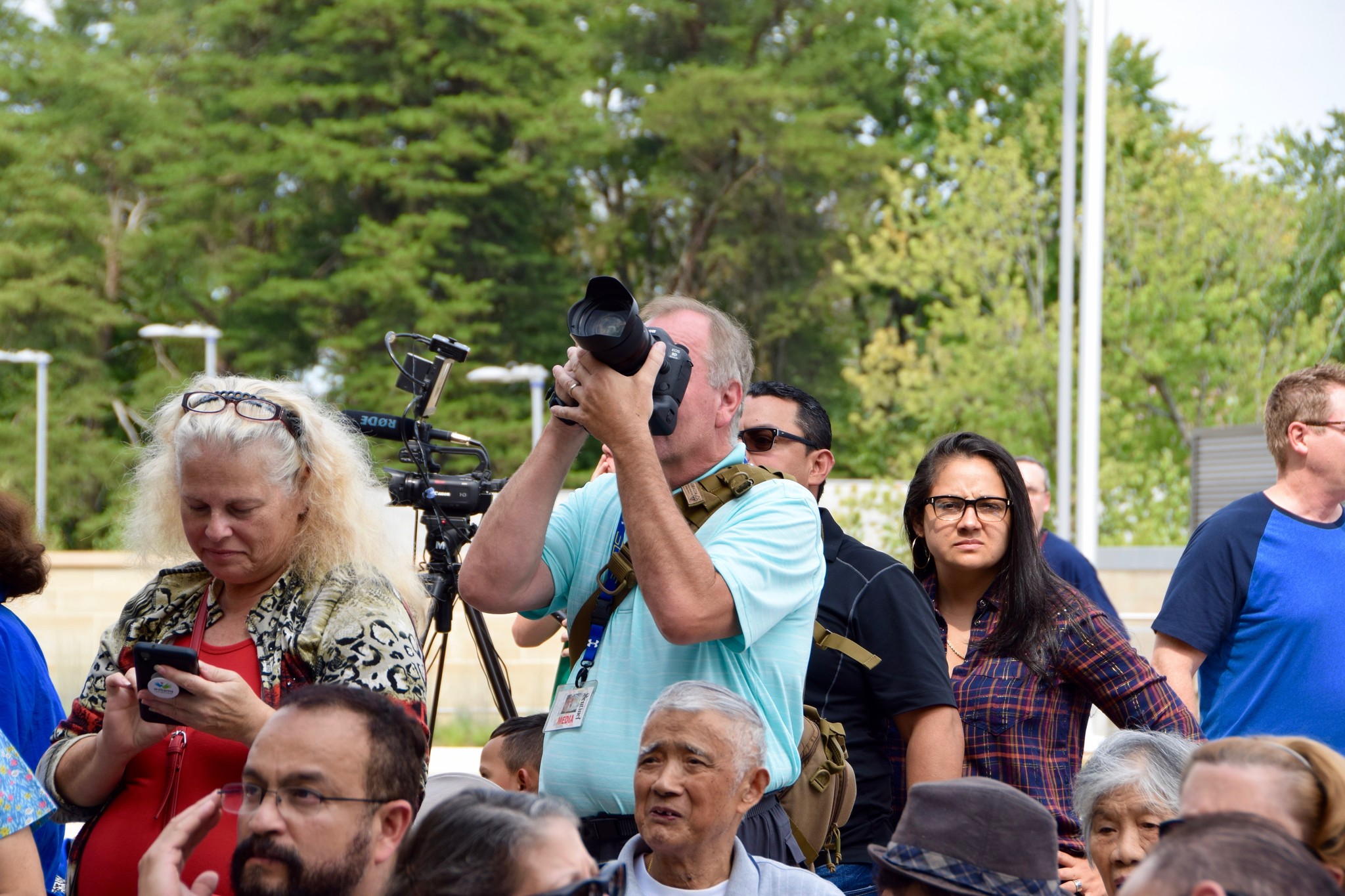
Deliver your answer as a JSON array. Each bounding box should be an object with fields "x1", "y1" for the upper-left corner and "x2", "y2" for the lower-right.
[{"x1": 776, "y1": 704, "x2": 858, "y2": 876}]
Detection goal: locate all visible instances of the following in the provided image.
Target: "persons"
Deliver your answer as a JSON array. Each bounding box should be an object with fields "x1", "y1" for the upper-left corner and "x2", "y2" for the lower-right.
[
  {"x1": 457, "y1": 297, "x2": 825, "y2": 867},
  {"x1": 1012, "y1": 455, "x2": 1130, "y2": 641},
  {"x1": 0, "y1": 488, "x2": 72, "y2": 896},
  {"x1": 1150, "y1": 361, "x2": 1345, "y2": 748},
  {"x1": 390, "y1": 787, "x2": 627, "y2": 896},
  {"x1": 599, "y1": 682, "x2": 847, "y2": 896},
  {"x1": 139, "y1": 685, "x2": 428, "y2": 896},
  {"x1": 901, "y1": 431, "x2": 1205, "y2": 896},
  {"x1": 34, "y1": 375, "x2": 433, "y2": 896},
  {"x1": 735, "y1": 382, "x2": 966, "y2": 896},
  {"x1": 867, "y1": 727, "x2": 1345, "y2": 896}
]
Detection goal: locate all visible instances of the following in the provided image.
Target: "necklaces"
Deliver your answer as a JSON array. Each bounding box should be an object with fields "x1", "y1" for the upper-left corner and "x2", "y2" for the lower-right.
[{"x1": 946, "y1": 638, "x2": 966, "y2": 661}]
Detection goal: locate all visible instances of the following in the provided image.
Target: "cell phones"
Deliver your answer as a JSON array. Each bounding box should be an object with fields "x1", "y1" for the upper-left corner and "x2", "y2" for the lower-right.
[{"x1": 134, "y1": 640, "x2": 200, "y2": 725}]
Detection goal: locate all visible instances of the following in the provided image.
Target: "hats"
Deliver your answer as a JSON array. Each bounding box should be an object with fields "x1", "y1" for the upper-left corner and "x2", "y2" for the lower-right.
[{"x1": 868, "y1": 776, "x2": 1075, "y2": 895}]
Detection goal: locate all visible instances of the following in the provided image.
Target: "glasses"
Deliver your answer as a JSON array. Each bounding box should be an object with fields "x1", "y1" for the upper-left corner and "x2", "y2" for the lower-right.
[
  {"x1": 737, "y1": 427, "x2": 820, "y2": 452},
  {"x1": 216, "y1": 781, "x2": 390, "y2": 819},
  {"x1": 181, "y1": 391, "x2": 311, "y2": 477},
  {"x1": 924, "y1": 495, "x2": 1013, "y2": 522},
  {"x1": 531, "y1": 859, "x2": 627, "y2": 896}
]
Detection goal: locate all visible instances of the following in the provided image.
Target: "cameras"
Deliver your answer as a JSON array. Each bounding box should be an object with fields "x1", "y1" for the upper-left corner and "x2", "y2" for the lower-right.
[{"x1": 541, "y1": 277, "x2": 694, "y2": 439}]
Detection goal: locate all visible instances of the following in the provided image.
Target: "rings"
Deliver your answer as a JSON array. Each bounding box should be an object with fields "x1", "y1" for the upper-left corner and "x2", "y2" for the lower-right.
[
  {"x1": 1072, "y1": 878, "x2": 1083, "y2": 892},
  {"x1": 570, "y1": 383, "x2": 581, "y2": 397}
]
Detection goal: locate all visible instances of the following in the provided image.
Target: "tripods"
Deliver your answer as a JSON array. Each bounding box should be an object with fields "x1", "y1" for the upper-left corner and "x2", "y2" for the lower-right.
[{"x1": 404, "y1": 522, "x2": 517, "y2": 774}]
